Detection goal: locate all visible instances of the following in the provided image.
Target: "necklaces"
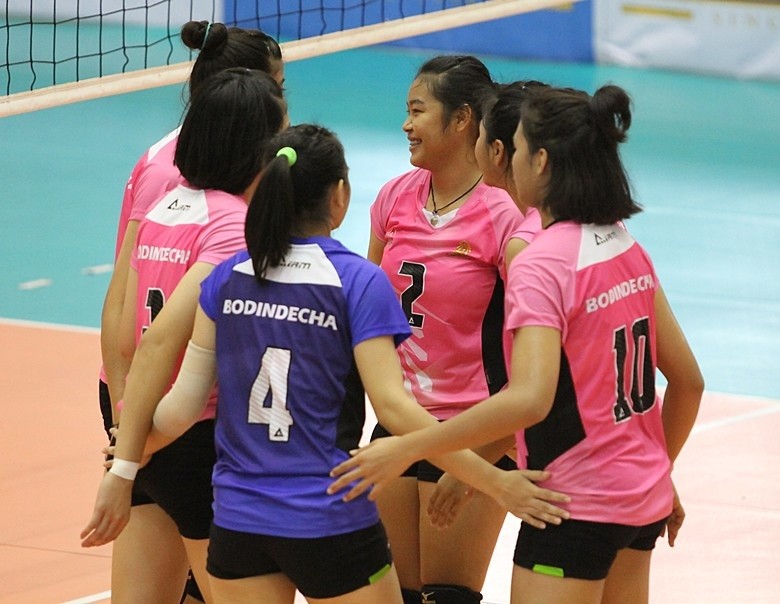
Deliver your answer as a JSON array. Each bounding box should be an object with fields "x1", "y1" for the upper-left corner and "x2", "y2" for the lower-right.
[{"x1": 429, "y1": 173, "x2": 483, "y2": 225}]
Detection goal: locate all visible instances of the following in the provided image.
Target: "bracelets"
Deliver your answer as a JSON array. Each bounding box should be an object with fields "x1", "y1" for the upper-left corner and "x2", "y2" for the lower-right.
[{"x1": 109, "y1": 458, "x2": 140, "y2": 480}]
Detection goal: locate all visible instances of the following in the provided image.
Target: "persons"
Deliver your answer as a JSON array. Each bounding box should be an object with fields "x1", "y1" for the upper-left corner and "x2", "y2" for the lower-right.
[
  {"x1": 360, "y1": 55, "x2": 527, "y2": 604},
  {"x1": 326, "y1": 84, "x2": 706, "y2": 604},
  {"x1": 102, "y1": 125, "x2": 571, "y2": 604},
  {"x1": 425, "y1": 78, "x2": 570, "y2": 528},
  {"x1": 81, "y1": 67, "x2": 292, "y2": 604},
  {"x1": 98, "y1": 20, "x2": 285, "y2": 604}
]
